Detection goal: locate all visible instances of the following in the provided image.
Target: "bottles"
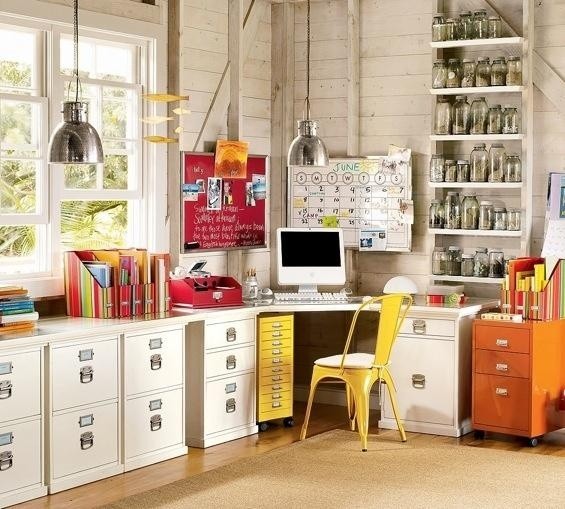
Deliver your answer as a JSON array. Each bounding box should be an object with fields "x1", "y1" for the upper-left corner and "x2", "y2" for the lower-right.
[
  {"x1": 432, "y1": 9, "x2": 502, "y2": 42},
  {"x1": 432, "y1": 57, "x2": 522, "y2": 89},
  {"x1": 435, "y1": 95, "x2": 519, "y2": 135},
  {"x1": 242, "y1": 277, "x2": 258, "y2": 305},
  {"x1": 430, "y1": 143, "x2": 521, "y2": 183},
  {"x1": 429, "y1": 191, "x2": 521, "y2": 230},
  {"x1": 432, "y1": 246, "x2": 516, "y2": 278}
]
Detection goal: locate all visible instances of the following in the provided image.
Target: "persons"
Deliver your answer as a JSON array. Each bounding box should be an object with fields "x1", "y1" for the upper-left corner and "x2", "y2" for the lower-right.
[{"x1": 223, "y1": 182, "x2": 233, "y2": 205}]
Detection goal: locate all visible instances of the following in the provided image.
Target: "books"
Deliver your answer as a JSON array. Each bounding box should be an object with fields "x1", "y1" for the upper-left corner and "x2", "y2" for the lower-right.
[
  {"x1": 502, "y1": 259, "x2": 550, "y2": 294},
  {"x1": 0, "y1": 287, "x2": 39, "y2": 341},
  {"x1": 83, "y1": 255, "x2": 169, "y2": 289}
]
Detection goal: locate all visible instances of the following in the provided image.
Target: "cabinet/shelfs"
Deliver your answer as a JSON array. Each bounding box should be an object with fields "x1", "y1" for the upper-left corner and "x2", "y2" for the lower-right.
[
  {"x1": 430, "y1": 36, "x2": 523, "y2": 285},
  {"x1": 1, "y1": 344, "x2": 51, "y2": 509},
  {"x1": 120, "y1": 323, "x2": 190, "y2": 472},
  {"x1": 472, "y1": 319, "x2": 565, "y2": 435},
  {"x1": 258, "y1": 312, "x2": 296, "y2": 431},
  {"x1": 46, "y1": 334, "x2": 123, "y2": 493}
]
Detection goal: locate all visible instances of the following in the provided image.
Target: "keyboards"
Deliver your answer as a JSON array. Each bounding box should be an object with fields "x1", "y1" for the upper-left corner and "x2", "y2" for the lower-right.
[{"x1": 273, "y1": 293, "x2": 348, "y2": 302}]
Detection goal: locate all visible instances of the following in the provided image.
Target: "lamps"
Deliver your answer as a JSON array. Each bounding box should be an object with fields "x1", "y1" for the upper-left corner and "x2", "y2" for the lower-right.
[
  {"x1": 47, "y1": 1, "x2": 103, "y2": 165},
  {"x1": 287, "y1": 0, "x2": 328, "y2": 169}
]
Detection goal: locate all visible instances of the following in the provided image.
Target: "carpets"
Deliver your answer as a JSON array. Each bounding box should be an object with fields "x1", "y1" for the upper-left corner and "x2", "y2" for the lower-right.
[{"x1": 90, "y1": 428, "x2": 564, "y2": 507}]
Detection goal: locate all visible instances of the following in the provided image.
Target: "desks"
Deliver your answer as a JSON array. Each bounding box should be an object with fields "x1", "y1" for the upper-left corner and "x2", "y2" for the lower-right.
[{"x1": 174, "y1": 293, "x2": 501, "y2": 449}]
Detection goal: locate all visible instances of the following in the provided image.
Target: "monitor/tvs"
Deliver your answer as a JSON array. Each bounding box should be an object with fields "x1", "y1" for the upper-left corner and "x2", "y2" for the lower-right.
[{"x1": 275, "y1": 225, "x2": 346, "y2": 293}]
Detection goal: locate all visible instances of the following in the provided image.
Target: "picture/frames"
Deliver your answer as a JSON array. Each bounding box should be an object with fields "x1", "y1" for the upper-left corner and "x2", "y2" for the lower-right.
[{"x1": 546, "y1": 172, "x2": 565, "y2": 224}]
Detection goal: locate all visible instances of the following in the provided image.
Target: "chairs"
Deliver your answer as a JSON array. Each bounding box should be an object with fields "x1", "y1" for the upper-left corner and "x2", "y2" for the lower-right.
[{"x1": 299, "y1": 292, "x2": 413, "y2": 451}]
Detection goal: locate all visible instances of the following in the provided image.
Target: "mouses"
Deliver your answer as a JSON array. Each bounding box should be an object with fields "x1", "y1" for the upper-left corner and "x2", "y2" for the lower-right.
[{"x1": 361, "y1": 294, "x2": 374, "y2": 306}]
